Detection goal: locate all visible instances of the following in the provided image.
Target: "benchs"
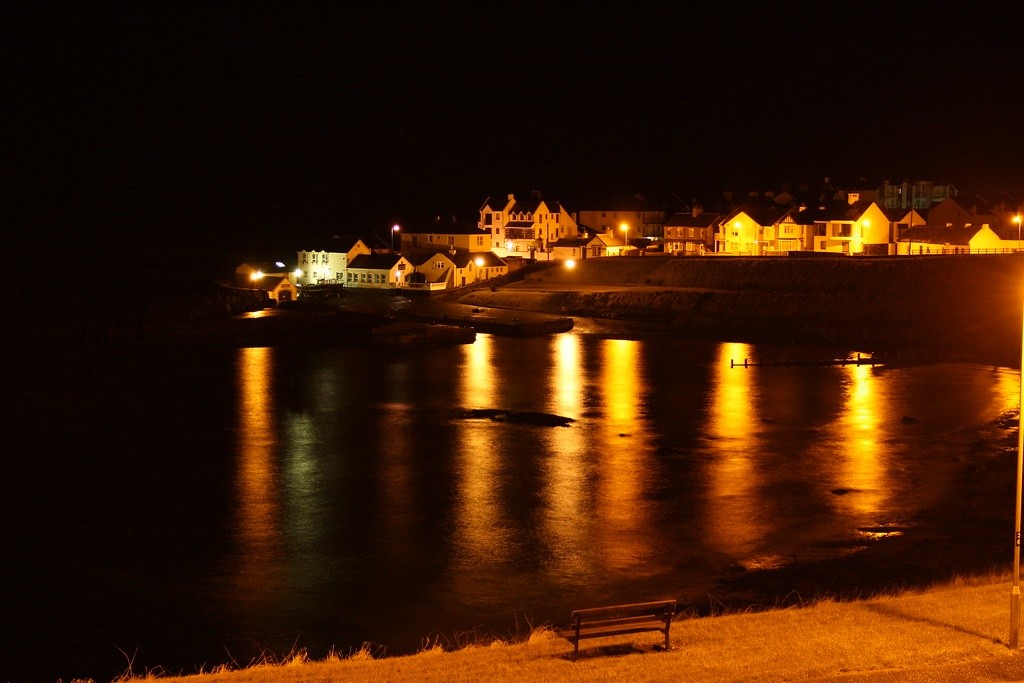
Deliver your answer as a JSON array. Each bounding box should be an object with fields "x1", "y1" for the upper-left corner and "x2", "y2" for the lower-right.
[{"x1": 561, "y1": 600, "x2": 677, "y2": 663}]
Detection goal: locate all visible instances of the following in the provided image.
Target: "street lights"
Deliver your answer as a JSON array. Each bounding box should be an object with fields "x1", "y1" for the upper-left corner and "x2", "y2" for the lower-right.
[
  {"x1": 391, "y1": 225, "x2": 400, "y2": 249},
  {"x1": 1014, "y1": 215, "x2": 1021, "y2": 248},
  {"x1": 622, "y1": 224, "x2": 628, "y2": 249}
]
